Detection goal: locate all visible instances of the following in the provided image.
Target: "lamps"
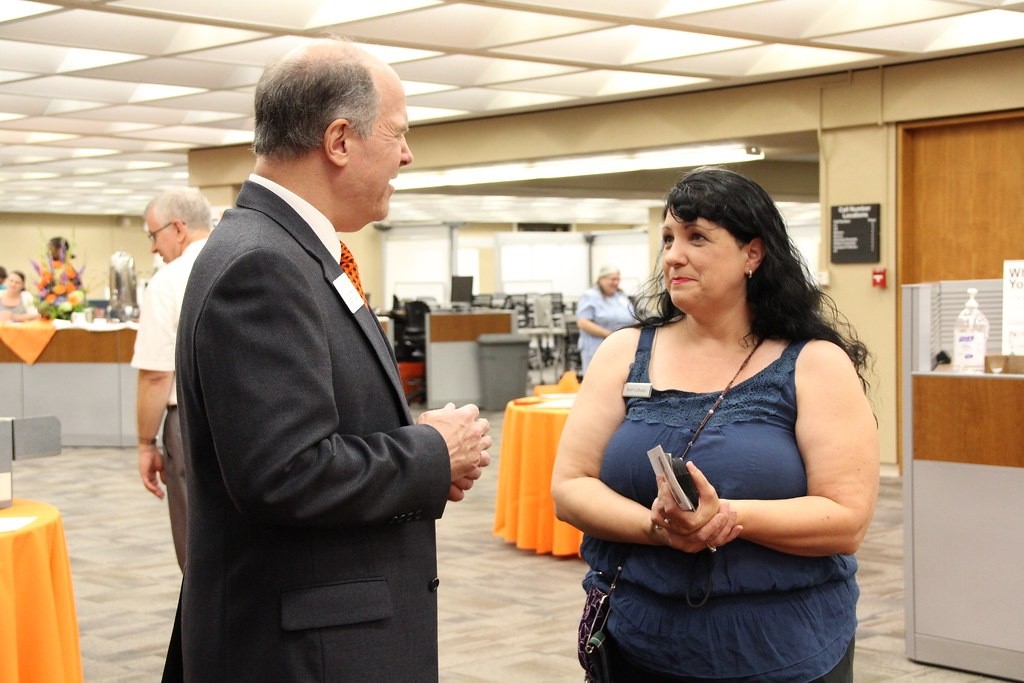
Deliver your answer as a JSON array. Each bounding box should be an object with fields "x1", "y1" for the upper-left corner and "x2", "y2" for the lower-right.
[{"x1": 389, "y1": 145, "x2": 760, "y2": 190}]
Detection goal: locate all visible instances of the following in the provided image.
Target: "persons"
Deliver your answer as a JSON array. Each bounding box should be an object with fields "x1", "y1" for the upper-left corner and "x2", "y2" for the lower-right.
[
  {"x1": 0, "y1": 266, "x2": 7, "y2": 290},
  {"x1": 131, "y1": 186, "x2": 217, "y2": 575},
  {"x1": 574, "y1": 262, "x2": 639, "y2": 376},
  {"x1": 47, "y1": 238, "x2": 75, "y2": 263},
  {"x1": 159, "y1": 41, "x2": 492, "y2": 683},
  {"x1": 0, "y1": 272, "x2": 41, "y2": 321},
  {"x1": 551, "y1": 164, "x2": 886, "y2": 683}
]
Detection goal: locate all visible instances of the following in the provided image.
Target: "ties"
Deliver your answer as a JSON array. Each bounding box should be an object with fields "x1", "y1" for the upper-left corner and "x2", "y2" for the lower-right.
[{"x1": 331, "y1": 239, "x2": 373, "y2": 311}]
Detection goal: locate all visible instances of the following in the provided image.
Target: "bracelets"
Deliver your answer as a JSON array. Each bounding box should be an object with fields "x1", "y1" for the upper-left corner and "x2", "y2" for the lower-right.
[{"x1": 140, "y1": 436, "x2": 156, "y2": 445}]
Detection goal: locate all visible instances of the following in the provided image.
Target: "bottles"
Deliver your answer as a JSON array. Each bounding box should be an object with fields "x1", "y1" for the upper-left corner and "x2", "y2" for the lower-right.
[{"x1": 952, "y1": 287, "x2": 988, "y2": 375}]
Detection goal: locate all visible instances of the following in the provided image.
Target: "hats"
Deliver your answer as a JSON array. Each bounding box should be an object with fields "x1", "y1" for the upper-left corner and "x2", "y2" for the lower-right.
[{"x1": 595, "y1": 264, "x2": 620, "y2": 279}]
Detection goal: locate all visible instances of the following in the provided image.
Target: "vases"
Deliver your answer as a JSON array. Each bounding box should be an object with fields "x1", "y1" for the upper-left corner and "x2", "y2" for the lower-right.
[{"x1": 40, "y1": 305, "x2": 78, "y2": 320}]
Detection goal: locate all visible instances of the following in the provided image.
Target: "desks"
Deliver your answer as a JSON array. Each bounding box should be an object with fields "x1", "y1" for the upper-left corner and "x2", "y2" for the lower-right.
[
  {"x1": 494, "y1": 395, "x2": 584, "y2": 560},
  {"x1": 0, "y1": 499, "x2": 86, "y2": 683}
]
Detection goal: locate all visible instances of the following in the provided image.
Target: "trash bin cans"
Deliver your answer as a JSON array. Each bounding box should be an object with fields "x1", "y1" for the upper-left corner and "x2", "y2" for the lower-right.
[{"x1": 476, "y1": 334, "x2": 532, "y2": 410}]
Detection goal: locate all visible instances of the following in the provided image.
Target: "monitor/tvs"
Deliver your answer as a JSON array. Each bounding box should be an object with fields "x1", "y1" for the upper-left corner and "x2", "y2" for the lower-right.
[{"x1": 450, "y1": 276, "x2": 472, "y2": 308}]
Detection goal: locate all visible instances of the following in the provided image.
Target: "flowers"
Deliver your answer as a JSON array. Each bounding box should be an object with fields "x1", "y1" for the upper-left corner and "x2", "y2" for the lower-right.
[{"x1": 27, "y1": 248, "x2": 87, "y2": 311}]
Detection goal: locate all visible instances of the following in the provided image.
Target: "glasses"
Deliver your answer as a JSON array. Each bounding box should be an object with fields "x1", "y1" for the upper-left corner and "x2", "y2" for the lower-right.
[{"x1": 148, "y1": 219, "x2": 188, "y2": 242}]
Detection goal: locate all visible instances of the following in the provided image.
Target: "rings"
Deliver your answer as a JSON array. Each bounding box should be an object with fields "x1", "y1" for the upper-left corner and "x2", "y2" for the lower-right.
[
  {"x1": 705, "y1": 542, "x2": 717, "y2": 552},
  {"x1": 663, "y1": 516, "x2": 671, "y2": 523},
  {"x1": 656, "y1": 524, "x2": 662, "y2": 530}
]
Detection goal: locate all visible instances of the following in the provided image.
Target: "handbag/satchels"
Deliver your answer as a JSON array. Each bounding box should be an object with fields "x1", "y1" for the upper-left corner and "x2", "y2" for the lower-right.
[{"x1": 576, "y1": 586, "x2": 612, "y2": 670}]
